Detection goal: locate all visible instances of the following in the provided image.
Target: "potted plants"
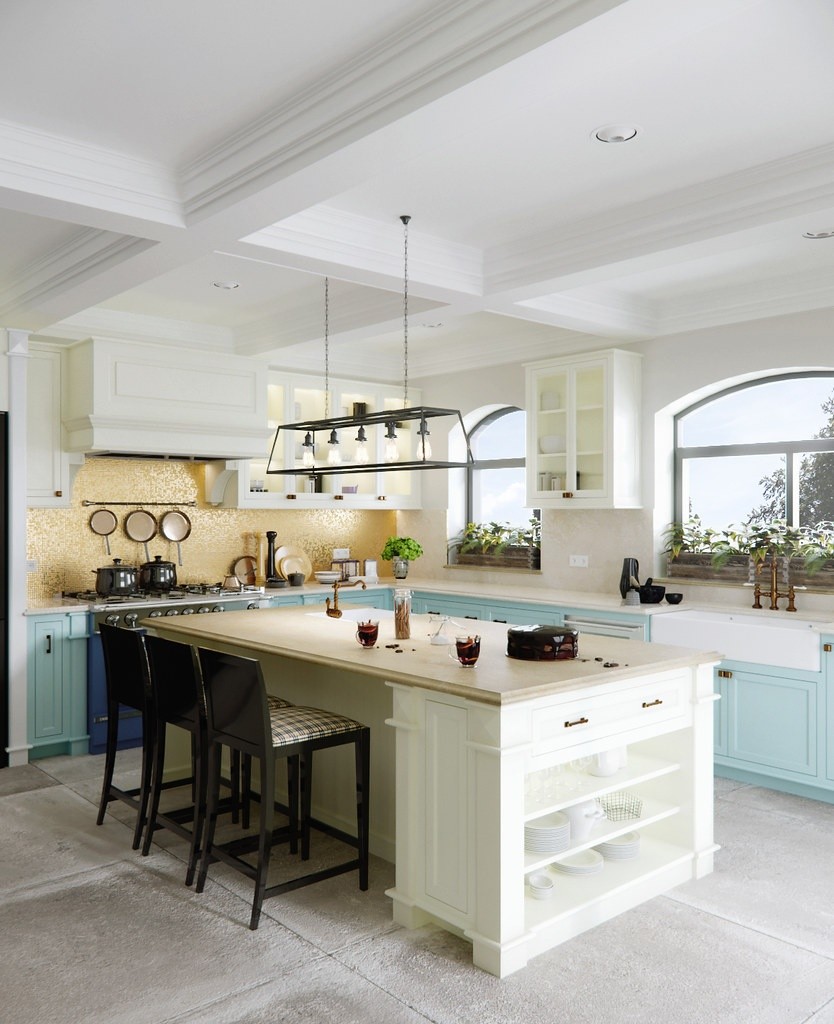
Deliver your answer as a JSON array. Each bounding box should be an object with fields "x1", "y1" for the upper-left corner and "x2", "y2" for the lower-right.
[
  {"x1": 381, "y1": 537, "x2": 423, "y2": 580},
  {"x1": 445, "y1": 521, "x2": 541, "y2": 570},
  {"x1": 660, "y1": 514, "x2": 834, "y2": 587}
]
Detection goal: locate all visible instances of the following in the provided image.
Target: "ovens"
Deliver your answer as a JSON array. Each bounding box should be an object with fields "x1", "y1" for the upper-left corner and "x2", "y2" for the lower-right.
[{"x1": 86, "y1": 601, "x2": 270, "y2": 755}]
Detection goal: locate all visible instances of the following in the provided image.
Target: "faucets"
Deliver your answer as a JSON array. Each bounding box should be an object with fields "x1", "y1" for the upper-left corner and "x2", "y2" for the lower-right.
[
  {"x1": 325, "y1": 579, "x2": 367, "y2": 619},
  {"x1": 752, "y1": 547, "x2": 797, "y2": 612}
]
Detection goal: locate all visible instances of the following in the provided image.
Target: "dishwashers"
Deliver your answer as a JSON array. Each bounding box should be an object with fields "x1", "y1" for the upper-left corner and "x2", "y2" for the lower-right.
[{"x1": 564, "y1": 616, "x2": 646, "y2": 644}]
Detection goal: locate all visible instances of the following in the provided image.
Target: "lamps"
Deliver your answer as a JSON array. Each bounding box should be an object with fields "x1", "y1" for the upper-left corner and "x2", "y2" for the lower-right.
[{"x1": 265, "y1": 215, "x2": 475, "y2": 476}]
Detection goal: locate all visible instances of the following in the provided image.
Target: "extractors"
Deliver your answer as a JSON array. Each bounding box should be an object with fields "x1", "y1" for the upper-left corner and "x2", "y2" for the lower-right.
[{"x1": 60, "y1": 337, "x2": 269, "y2": 467}]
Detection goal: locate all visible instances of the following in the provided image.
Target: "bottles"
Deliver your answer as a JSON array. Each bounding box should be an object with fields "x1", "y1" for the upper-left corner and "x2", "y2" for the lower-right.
[
  {"x1": 429, "y1": 613, "x2": 450, "y2": 645},
  {"x1": 394, "y1": 589, "x2": 412, "y2": 639},
  {"x1": 619, "y1": 558, "x2": 640, "y2": 605}
]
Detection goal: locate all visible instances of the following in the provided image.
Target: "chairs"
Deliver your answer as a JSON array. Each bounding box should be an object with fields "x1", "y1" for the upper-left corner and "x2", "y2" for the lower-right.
[{"x1": 96, "y1": 623, "x2": 371, "y2": 930}]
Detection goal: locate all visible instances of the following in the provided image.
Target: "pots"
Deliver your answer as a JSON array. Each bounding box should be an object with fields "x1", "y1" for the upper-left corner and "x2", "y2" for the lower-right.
[
  {"x1": 139, "y1": 556, "x2": 177, "y2": 590},
  {"x1": 90, "y1": 510, "x2": 118, "y2": 555},
  {"x1": 160, "y1": 510, "x2": 192, "y2": 565},
  {"x1": 91, "y1": 558, "x2": 137, "y2": 596},
  {"x1": 126, "y1": 510, "x2": 158, "y2": 562}
]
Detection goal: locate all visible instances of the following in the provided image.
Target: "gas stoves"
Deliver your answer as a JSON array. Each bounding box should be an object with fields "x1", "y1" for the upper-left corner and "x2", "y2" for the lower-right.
[{"x1": 62, "y1": 582, "x2": 272, "y2": 612}]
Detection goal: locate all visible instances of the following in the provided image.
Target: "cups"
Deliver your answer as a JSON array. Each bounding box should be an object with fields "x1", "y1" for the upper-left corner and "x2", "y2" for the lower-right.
[
  {"x1": 355, "y1": 620, "x2": 379, "y2": 648},
  {"x1": 447, "y1": 636, "x2": 481, "y2": 668},
  {"x1": 589, "y1": 750, "x2": 619, "y2": 776},
  {"x1": 542, "y1": 392, "x2": 559, "y2": 411}
]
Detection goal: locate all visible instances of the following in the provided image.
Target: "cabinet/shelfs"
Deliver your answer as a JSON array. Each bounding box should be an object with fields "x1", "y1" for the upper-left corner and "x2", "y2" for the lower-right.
[
  {"x1": 23, "y1": 342, "x2": 71, "y2": 509},
  {"x1": 410, "y1": 592, "x2": 566, "y2": 629},
  {"x1": 523, "y1": 347, "x2": 642, "y2": 510},
  {"x1": 712, "y1": 659, "x2": 820, "y2": 801},
  {"x1": 205, "y1": 369, "x2": 423, "y2": 510},
  {"x1": 139, "y1": 604, "x2": 723, "y2": 979},
  {"x1": 820, "y1": 633, "x2": 834, "y2": 806},
  {"x1": 24, "y1": 611, "x2": 90, "y2": 761},
  {"x1": 272, "y1": 589, "x2": 393, "y2": 612}
]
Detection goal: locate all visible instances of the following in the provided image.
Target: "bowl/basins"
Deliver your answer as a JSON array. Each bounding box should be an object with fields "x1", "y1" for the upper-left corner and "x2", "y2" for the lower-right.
[
  {"x1": 342, "y1": 486, "x2": 357, "y2": 493},
  {"x1": 632, "y1": 586, "x2": 683, "y2": 604},
  {"x1": 528, "y1": 875, "x2": 553, "y2": 900},
  {"x1": 288, "y1": 572, "x2": 305, "y2": 586},
  {"x1": 315, "y1": 571, "x2": 343, "y2": 584},
  {"x1": 540, "y1": 434, "x2": 566, "y2": 454}
]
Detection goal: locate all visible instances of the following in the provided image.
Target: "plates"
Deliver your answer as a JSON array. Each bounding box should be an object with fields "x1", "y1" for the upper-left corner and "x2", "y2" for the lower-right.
[
  {"x1": 349, "y1": 576, "x2": 378, "y2": 583},
  {"x1": 274, "y1": 544, "x2": 312, "y2": 582},
  {"x1": 235, "y1": 556, "x2": 257, "y2": 584},
  {"x1": 523, "y1": 812, "x2": 643, "y2": 876}
]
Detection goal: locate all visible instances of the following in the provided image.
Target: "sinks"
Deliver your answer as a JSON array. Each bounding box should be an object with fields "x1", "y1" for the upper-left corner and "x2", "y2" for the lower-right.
[
  {"x1": 650, "y1": 609, "x2": 826, "y2": 672},
  {"x1": 307, "y1": 608, "x2": 414, "y2": 623}
]
